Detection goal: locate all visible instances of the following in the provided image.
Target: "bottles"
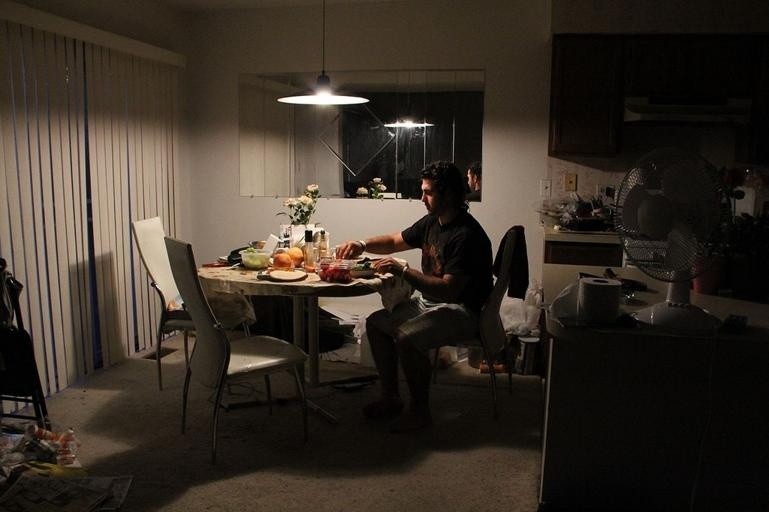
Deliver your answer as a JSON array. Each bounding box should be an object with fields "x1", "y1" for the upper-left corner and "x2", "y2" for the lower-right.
[
  {"x1": 304, "y1": 230, "x2": 316, "y2": 272},
  {"x1": 319, "y1": 230, "x2": 327, "y2": 261}
]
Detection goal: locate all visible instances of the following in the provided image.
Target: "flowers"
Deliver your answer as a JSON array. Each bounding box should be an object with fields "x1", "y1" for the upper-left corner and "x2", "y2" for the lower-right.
[
  {"x1": 275, "y1": 184, "x2": 322, "y2": 225},
  {"x1": 356, "y1": 176, "x2": 387, "y2": 201}
]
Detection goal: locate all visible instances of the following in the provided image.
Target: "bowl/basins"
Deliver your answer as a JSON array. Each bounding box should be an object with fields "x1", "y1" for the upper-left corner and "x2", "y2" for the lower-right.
[{"x1": 239, "y1": 249, "x2": 273, "y2": 268}]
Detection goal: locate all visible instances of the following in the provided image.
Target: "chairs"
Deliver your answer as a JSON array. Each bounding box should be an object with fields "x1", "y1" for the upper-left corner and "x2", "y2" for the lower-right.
[
  {"x1": 432, "y1": 224, "x2": 530, "y2": 420},
  {"x1": 0, "y1": 276, "x2": 53, "y2": 434},
  {"x1": 163, "y1": 236, "x2": 310, "y2": 465},
  {"x1": 131, "y1": 215, "x2": 250, "y2": 395}
]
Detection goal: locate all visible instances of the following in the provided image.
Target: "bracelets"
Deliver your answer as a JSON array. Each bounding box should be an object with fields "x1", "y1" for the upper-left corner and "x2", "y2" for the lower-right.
[
  {"x1": 356, "y1": 240, "x2": 366, "y2": 248},
  {"x1": 401, "y1": 265, "x2": 408, "y2": 278}
]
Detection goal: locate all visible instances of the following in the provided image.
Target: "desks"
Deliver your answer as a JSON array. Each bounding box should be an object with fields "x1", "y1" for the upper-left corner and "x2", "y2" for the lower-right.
[{"x1": 538, "y1": 263, "x2": 769, "y2": 512}]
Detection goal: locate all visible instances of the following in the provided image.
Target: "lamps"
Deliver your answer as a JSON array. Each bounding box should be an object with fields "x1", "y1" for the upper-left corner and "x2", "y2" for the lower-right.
[
  {"x1": 383, "y1": 111, "x2": 436, "y2": 129},
  {"x1": 276, "y1": 74, "x2": 370, "y2": 106}
]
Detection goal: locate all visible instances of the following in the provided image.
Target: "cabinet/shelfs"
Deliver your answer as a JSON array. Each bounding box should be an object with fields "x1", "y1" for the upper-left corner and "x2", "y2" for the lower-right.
[{"x1": 547, "y1": 33, "x2": 768, "y2": 158}]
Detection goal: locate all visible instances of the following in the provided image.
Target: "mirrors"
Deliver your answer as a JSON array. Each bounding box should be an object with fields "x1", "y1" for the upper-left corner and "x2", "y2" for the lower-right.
[{"x1": 237, "y1": 69, "x2": 486, "y2": 203}]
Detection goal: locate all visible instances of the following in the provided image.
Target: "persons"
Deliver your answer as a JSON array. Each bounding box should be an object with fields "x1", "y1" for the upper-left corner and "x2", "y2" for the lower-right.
[
  {"x1": 335, "y1": 160, "x2": 495, "y2": 431},
  {"x1": 464, "y1": 161, "x2": 483, "y2": 201}
]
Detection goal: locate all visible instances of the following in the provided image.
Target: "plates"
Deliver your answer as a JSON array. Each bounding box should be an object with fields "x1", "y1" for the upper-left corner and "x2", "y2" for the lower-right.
[{"x1": 351, "y1": 269, "x2": 375, "y2": 277}]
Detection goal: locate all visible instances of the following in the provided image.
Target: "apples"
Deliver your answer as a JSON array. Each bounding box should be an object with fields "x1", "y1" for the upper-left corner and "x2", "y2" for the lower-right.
[
  {"x1": 286, "y1": 247, "x2": 304, "y2": 267},
  {"x1": 273, "y1": 253, "x2": 291, "y2": 269}
]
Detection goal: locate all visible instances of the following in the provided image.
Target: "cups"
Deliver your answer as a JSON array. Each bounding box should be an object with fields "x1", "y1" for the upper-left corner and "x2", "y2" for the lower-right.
[{"x1": 321, "y1": 240, "x2": 335, "y2": 264}]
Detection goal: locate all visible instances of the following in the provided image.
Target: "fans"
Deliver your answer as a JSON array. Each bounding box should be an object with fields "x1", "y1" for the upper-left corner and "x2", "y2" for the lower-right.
[{"x1": 613, "y1": 145, "x2": 732, "y2": 332}]
